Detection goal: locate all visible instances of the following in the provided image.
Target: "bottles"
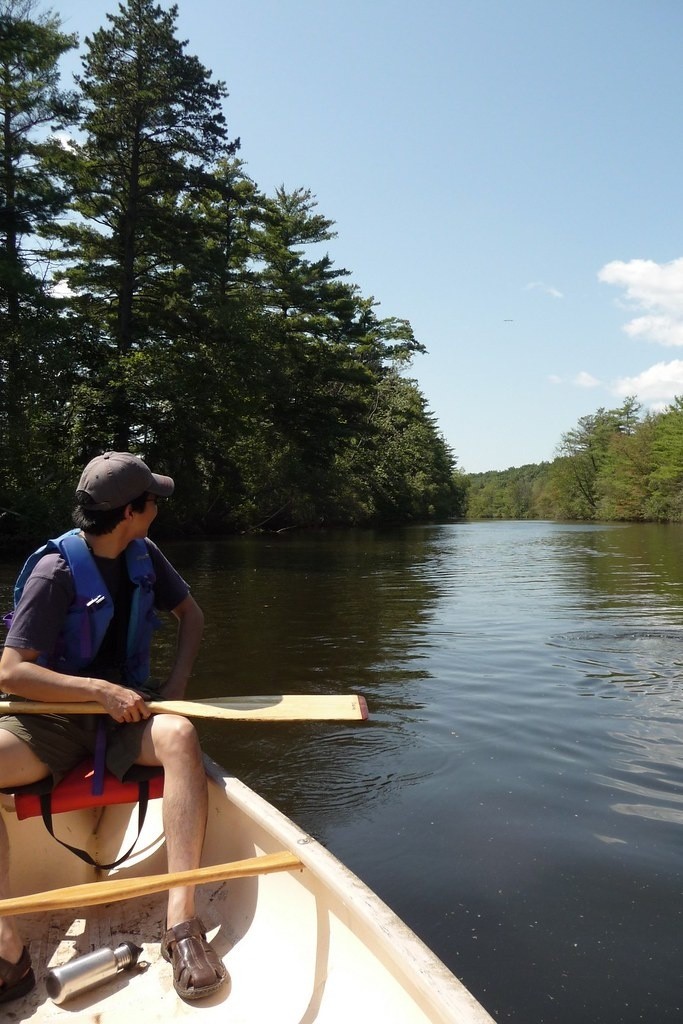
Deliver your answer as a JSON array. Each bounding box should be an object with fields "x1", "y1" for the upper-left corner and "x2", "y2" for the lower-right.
[{"x1": 45, "y1": 940, "x2": 143, "y2": 1005}]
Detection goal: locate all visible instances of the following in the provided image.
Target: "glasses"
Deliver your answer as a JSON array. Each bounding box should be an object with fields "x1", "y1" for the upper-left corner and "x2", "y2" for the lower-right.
[{"x1": 142, "y1": 495, "x2": 169, "y2": 507}]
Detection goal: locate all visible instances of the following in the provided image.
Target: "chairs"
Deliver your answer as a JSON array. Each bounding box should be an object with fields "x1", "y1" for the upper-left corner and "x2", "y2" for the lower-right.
[{"x1": 0, "y1": 764, "x2": 165, "y2": 824}]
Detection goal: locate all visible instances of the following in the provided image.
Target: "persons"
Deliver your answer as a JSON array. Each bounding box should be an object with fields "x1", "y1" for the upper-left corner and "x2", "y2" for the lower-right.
[{"x1": 1, "y1": 452, "x2": 228, "y2": 1004}]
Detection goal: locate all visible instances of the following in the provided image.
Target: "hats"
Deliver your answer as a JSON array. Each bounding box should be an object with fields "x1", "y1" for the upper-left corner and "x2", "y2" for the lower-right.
[{"x1": 74, "y1": 452, "x2": 175, "y2": 511}]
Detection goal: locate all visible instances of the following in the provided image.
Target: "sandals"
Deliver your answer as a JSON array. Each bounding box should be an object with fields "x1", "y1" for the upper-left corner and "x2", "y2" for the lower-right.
[
  {"x1": 0, "y1": 945, "x2": 35, "y2": 1003},
  {"x1": 160, "y1": 916, "x2": 227, "y2": 1000}
]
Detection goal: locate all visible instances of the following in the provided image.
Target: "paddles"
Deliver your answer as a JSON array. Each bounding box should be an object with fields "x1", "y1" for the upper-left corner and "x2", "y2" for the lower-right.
[{"x1": 0, "y1": 692, "x2": 372, "y2": 725}]
